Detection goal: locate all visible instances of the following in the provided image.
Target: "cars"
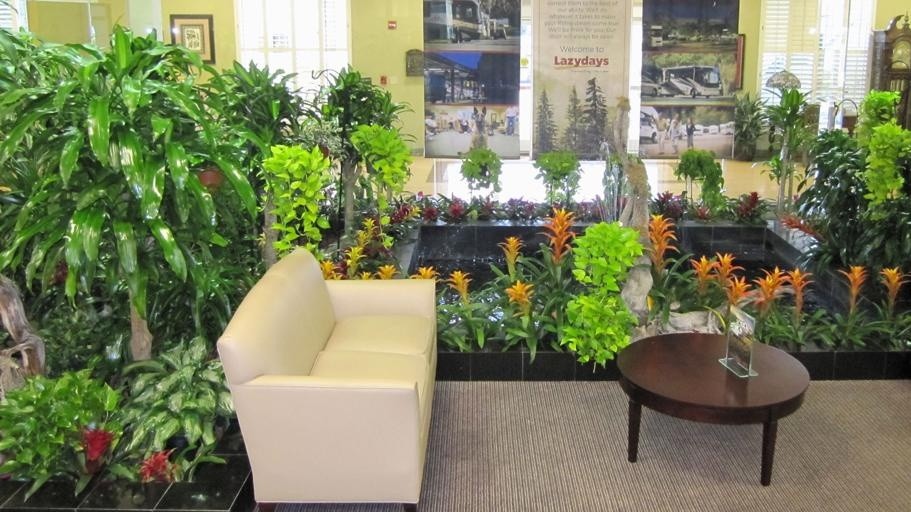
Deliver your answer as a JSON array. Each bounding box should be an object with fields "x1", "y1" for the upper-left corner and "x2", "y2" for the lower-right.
[
  {"x1": 640, "y1": 111, "x2": 660, "y2": 144},
  {"x1": 642, "y1": 71, "x2": 676, "y2": 98}
]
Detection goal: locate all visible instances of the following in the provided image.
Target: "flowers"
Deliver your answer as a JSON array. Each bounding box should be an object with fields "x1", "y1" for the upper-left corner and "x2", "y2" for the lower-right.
[
  {"x1": 638, "y1": 189, "x2": 850, "y2": 350},
  {"x1": 320, "y1": 218, "x2": 438, "y2": 280},
  {"x1": 390, "y1": 192, "x2": 629, "y2": 222},
  {"x1": 440, "y1": 207, "x2": 582, "y2": 364}
]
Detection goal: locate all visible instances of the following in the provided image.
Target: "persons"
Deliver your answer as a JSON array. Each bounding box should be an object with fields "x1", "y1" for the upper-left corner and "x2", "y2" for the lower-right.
[
  {"x1": 505, "y1": 103, "x2": 517, "y2": 134},
  {"x1": 652, "y1": 112, "x2": 695, "y2": 154}
]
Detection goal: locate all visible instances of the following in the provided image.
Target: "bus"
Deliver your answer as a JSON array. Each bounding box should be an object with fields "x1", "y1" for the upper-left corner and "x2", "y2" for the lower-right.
[
  {"x1": 643, "y1": 25, "x2": 664, "y2": 48},
  {"x1": 658, "y1": 65, "x2": 724, "y2": 99}
]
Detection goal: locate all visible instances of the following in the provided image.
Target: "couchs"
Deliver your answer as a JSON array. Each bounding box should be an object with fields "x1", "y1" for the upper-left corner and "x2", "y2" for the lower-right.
[{"x1": 216, "y1": 246, "x2": 439, "y2": 512}]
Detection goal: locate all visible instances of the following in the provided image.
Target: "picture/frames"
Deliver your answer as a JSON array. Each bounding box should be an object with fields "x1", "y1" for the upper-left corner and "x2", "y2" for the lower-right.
[{"x1": 169, "y1": 14, "x2": 216, "y2": 63}]
